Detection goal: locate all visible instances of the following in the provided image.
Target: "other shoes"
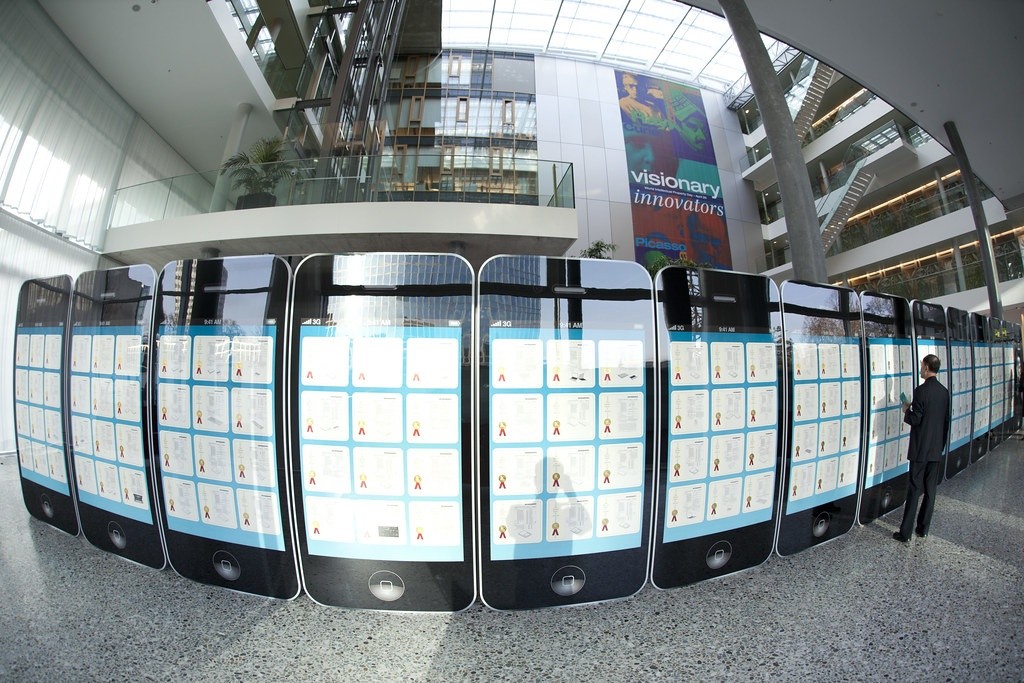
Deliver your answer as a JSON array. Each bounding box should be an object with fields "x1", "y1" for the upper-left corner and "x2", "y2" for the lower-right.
[
  {"x1": 894, "y1": 532, "x2": 911, "y2": 542},
  {"x1": 916, "y1": 527, "x2": 939, "y2": 534}
]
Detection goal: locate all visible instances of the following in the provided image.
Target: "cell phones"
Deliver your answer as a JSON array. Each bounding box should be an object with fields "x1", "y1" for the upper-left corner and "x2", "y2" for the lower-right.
[{"x1": 12, "y1": 251, "x2": 1024, "y2": 613}]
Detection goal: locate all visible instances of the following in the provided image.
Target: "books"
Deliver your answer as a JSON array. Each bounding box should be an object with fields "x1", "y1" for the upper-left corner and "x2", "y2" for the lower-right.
[{"x1": 666, "y1": 91, "x2": 706, "y2": 121}]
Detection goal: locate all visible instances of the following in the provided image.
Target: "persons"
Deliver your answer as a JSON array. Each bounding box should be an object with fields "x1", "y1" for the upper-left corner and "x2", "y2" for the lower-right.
[
  {"x1": 618, "y1": 74, "x2": 716, "y2": 165},
  {"x1": 893, "y1": 354, "x2": 951, "y2": 542}
]
